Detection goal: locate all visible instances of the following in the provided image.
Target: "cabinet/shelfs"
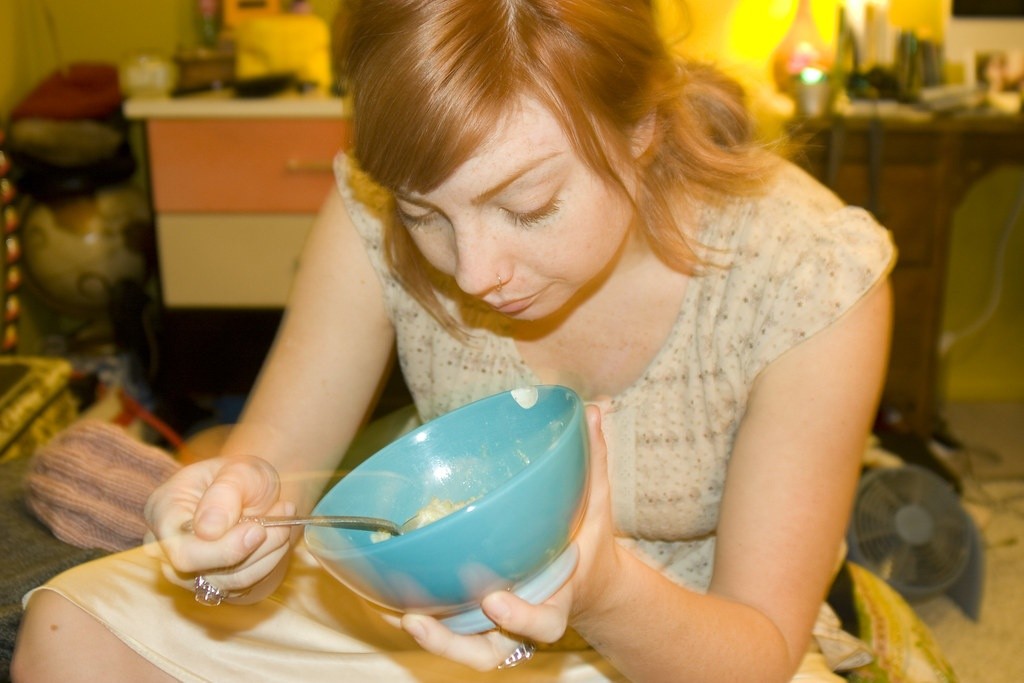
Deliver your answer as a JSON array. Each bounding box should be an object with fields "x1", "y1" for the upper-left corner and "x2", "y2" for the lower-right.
[{"x1": 129, "y1": 77, "x2": 410, "y2": 408}]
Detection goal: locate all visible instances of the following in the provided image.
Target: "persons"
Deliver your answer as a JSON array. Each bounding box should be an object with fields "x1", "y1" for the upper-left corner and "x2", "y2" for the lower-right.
[{"x1": 10, "y1": 1, "x2": 898, "y2": 683}]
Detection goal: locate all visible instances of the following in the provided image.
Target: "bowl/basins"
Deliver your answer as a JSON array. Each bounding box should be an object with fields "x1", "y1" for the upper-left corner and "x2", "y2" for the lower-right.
[{"x1": 302, "y1": 384, "x2": 592, "y2": 635}]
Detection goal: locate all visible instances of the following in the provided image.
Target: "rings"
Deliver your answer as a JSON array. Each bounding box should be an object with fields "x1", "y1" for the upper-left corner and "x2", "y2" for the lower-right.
[
  {"x1": 498, "y1": 634, "x2": 536, "y2": 671},
  {"x1": 190, "y1": 575, "x2": 229, "y2": 608}
]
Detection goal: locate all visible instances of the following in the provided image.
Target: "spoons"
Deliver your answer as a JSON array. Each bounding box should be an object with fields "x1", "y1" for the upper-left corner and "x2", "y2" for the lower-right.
[{"x1": 179, "y1": 512, "x2": 423, "y2": 537}]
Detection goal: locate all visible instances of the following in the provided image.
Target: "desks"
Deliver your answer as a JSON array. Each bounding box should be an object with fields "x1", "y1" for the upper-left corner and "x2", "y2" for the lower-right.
[{"x1": 773, "y1": 106, "x2": 1024, "y2": 457}]
